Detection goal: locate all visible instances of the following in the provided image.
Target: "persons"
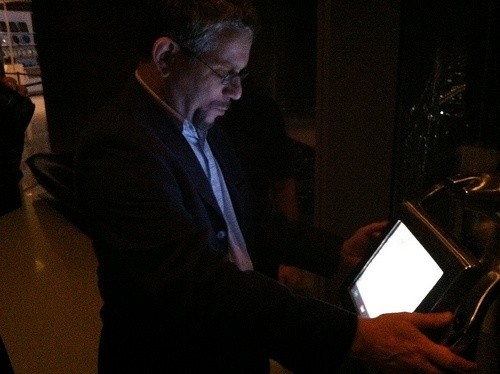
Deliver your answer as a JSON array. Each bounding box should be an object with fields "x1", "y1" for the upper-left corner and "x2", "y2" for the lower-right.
[{"x1": 73, "y1": 0, "x2": 478, "y2": 374}]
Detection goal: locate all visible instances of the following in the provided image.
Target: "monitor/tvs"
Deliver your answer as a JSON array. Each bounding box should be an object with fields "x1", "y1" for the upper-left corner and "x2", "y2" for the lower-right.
[{"x1": 339, "y1": 200, "x2": 486, "y2": 348}]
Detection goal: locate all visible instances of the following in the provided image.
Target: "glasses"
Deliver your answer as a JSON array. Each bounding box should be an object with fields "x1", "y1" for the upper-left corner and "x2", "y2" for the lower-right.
[{"x1": 172, "y1": 37, "x2": 249, "y2": 85}]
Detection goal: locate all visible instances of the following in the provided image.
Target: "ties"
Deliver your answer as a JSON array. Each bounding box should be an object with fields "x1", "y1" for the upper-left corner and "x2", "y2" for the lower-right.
[{"x1": 195, "y1": 129, "x2": 227, "y2": 213}]
